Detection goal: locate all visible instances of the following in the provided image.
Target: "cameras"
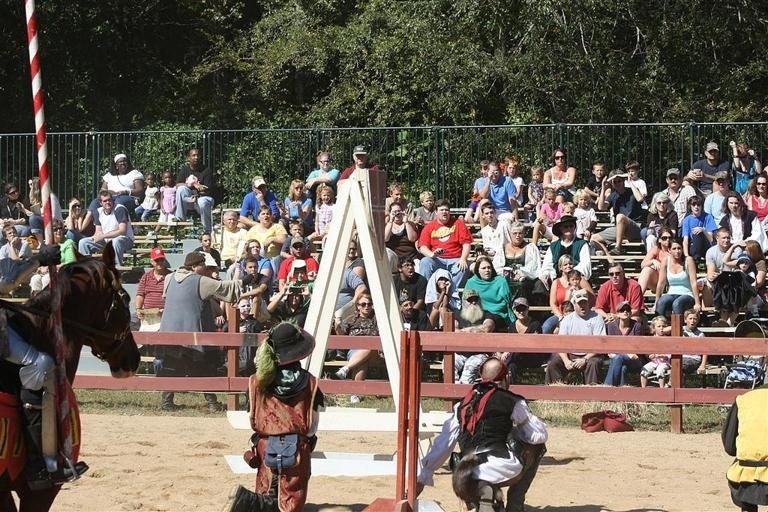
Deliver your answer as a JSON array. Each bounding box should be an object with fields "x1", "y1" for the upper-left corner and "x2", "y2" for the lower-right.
[
  {"x1": 395, "y1": 212, "x2": 401, "y2": 216},
  {"x1": 493, "y1": 171, "x2": 499, "y2": 175},
  {"x1": 290, "y1": 287, "x2": 303, "y2": 293}
]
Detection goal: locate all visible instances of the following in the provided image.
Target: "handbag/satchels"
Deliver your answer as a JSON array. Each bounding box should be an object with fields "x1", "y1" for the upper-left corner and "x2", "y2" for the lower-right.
[{"x1": 265, "y1": 434, "x2": 298, "y2": 468}]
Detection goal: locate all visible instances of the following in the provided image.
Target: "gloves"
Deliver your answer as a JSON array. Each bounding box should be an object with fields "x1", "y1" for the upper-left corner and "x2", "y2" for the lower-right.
[{"x1": 38, "y1": 244, "x2": 61, "y2": 266}]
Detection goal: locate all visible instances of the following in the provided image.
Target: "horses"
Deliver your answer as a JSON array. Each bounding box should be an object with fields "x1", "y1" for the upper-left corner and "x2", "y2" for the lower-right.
[{"x1": 0, "y1": 239, "x2": 141, "y2": 512}]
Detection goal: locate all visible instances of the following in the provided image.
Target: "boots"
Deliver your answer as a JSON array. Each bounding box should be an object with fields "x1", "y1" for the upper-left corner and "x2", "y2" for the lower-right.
[
  {"x1": 467, "y1": 480, "x2": 503, "y2": 512},
  {"x1": 162, "y1": 393, "x2": 185, "y2": 411},
  {"x1": 506, "y1": 440, "x2": 546, "y2": 511},
  {"x1": 204, "y1": 392, "x2": 227, "y2": 412},
  {"x1": 221, "y1": 484, "x2": 278, "y2": 512}
]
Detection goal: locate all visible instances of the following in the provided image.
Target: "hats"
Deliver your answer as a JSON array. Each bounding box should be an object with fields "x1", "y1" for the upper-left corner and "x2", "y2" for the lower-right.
[
  {"x1": 20, "y1": 386, "x2": 88, "y2": 491},
  {"x1": 291, "y1": 238, "x2": 304, "y2": 246},
  {"x1": 150, "y1": 246, "x2": 205, "y2": 269},
  {"x1": 464, "y1": 289, "x2": 479, "y2": 299},
  {"x1": 736, "y1": 254, "x2": 752, "y2": 266},
  {"x1": 552, "y1": 215, "x2": 577, "y2": 236},
  {"x1": 252, "y1": 176, "x2": 266, "y2": 188},
  {"x1": 616, "y1": 302, "x2": 631, "y2": 312},
  {"x1": 666, "y1": 168, "x2": 680, "y2": 177},
  {"x1": 353, "y1": 144, "x2": 367, "y2": 154},
  {"x1": 266, "y1": 323, "x2": 315, "y2": 366},
  {"x1": 705, "y1": 141, "x2": 727, "y2": 181},
  {"x1": 512, "y1": 297, "x2": 528, "y2": 309},
  {"x1": 574, "y1": 289, "x2": 588, "y2": 303}
]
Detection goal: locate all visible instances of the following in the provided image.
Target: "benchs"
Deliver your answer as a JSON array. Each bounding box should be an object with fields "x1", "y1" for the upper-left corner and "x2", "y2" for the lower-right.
[{"x1": 1, "y1": 199, "x2": 768, "y2": 397}]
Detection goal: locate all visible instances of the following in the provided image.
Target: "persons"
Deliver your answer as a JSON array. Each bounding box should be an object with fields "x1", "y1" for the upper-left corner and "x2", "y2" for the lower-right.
[
  {"x1": 243, "y1": 319, "x2": 324, "y2": 511},
  {"x1": 1, "y1": 244, "x2": 89, "y2": 491},
  {"x1": 722, "y1": 383, "x2": 768, "y2": 512},
  {"x1": 0, "y1": 143, "x2": 768, "y2": 403},
  {"x1": 403, "y1": 357, "x2": 549, "y2": 511}
]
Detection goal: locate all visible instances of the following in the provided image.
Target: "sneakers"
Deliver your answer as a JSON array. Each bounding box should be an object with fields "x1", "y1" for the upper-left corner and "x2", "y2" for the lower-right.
[{"x1": 335, "y1": 366, "x2": 349, "y2": 380}]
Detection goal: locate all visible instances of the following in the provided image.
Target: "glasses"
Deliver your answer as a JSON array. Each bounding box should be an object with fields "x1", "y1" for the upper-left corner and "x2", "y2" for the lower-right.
[
  {"x1": 358, "y1": 303, "x2": 372, "y2": 306},
  {"x1": 554, "y1": 156, "x2": 565, "y2": 159},
  {"x1": 661, "y1": 236, "x2": 672, "y2": 241},
  {"x1": 610, "y1": 272, "x2": 620, "y2": 275}
]
